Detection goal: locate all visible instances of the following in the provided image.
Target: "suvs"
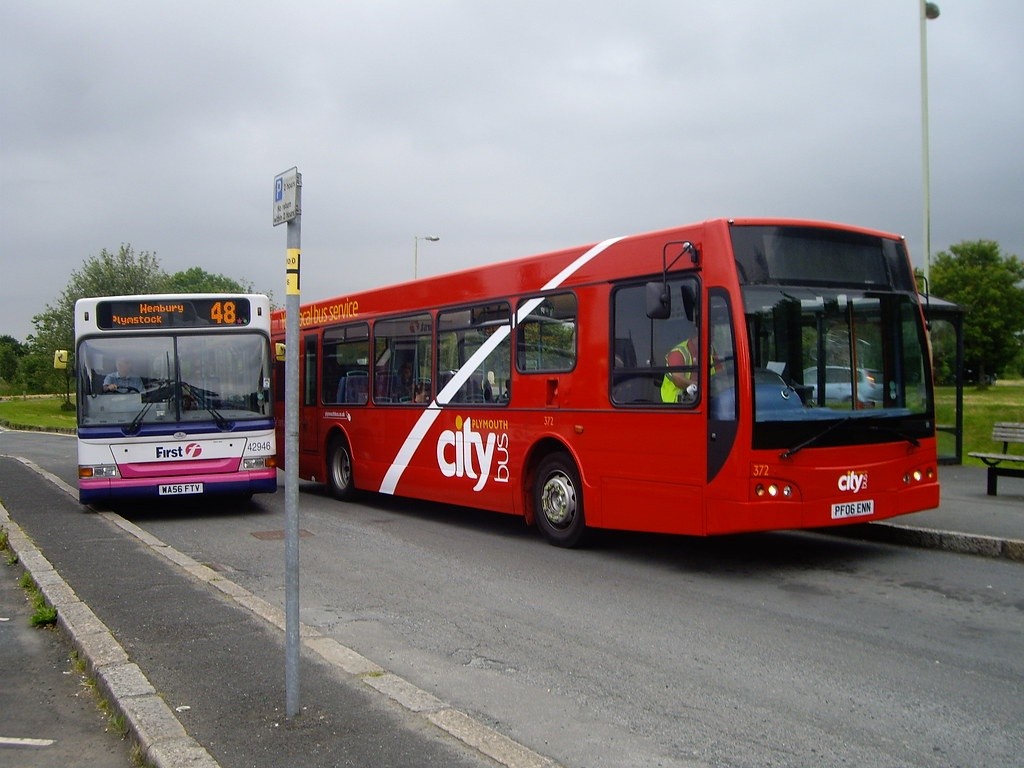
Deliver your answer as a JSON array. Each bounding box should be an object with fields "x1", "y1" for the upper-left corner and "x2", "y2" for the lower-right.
[{"x1": 799, "y1": 363, "x2": 895, "y2": 404}]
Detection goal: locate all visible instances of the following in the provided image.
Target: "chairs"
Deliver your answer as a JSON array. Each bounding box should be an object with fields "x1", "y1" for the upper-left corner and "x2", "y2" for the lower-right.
[{"x1": 336, "y1": 369, "x2": 493, "y2": 403}]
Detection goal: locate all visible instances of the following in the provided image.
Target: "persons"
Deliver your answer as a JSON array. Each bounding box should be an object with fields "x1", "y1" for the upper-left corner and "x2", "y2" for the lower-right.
[
  {"x1": 102, "y1": 354, "x2": 145, "y2": 405},
  {"x1": 660, "y1": 324, "x2": 724, "y2": 404},
  {"x1": 496, "y1": 379, "x2": 510, "y2": 402},
  {"x1": 393, "y1": 362, "x2": 418, "y2": 400},
  {"x1": 402, "y1": 384, "x2": 431, "y2": 404}
]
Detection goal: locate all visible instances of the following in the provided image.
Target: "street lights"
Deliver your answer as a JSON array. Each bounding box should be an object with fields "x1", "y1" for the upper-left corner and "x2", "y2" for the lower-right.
[
  {"x1": 414, "y1": 235, "x2": 439, "y2": 279},
  {"x1": 919, "y1": 1, "x2": 941, "y2": 296}
]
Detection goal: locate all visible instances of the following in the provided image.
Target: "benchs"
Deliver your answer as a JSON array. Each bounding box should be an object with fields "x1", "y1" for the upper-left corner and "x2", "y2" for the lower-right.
[{"x1": 967, "y1": 422, "x2": 1024, "y2": 496}]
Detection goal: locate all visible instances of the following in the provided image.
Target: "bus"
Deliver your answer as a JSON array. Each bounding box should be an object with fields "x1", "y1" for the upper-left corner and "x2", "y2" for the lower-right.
[
  {"x1": 270, "y1": 216, "x2": 941, "y2": 547},
  {"x1": 54, "y1": 294, "x2": 286, "y2": 510}
]
